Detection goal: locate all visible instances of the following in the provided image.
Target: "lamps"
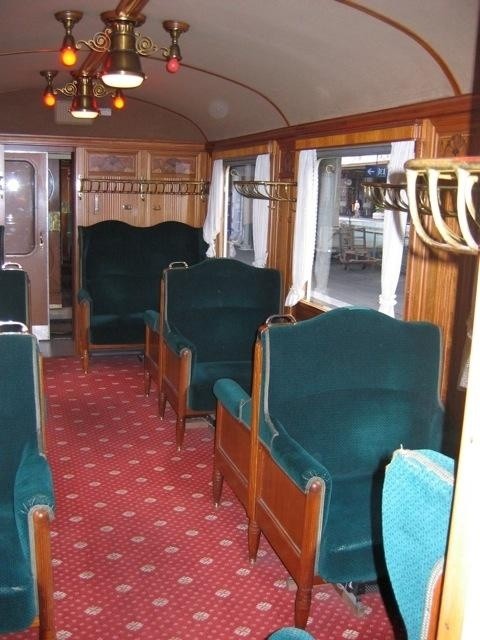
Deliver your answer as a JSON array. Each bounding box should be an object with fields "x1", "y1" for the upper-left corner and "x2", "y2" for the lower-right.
[{"x1": 41, "y1": 0, "x2": 188, "y2": 119}]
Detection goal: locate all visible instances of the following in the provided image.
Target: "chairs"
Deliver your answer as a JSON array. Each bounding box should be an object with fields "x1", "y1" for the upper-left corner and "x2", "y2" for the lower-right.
[
  {"x1": 0, "y1": 261, "x2": 57, "y2": 640},
  {"x1": 213, "y1": 305, "x2": 459, "y2": 631},
  {"x1": 141, "y1": 256, "x2": 296, "y2": 452}
]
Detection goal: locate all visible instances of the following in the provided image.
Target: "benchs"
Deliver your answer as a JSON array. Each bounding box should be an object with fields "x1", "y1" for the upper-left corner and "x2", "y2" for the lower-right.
[{"x1": 72, "y1": 221, "x2": 217, "y2": 374}]
